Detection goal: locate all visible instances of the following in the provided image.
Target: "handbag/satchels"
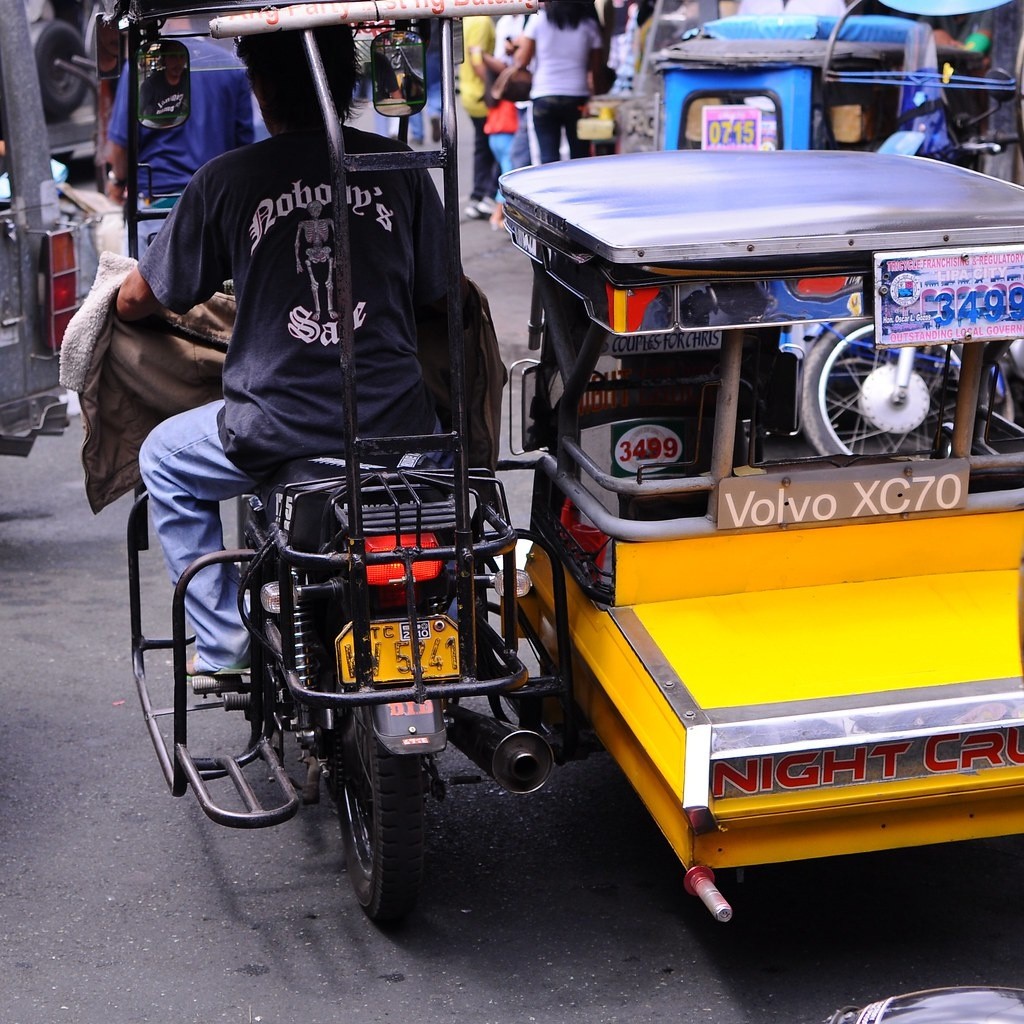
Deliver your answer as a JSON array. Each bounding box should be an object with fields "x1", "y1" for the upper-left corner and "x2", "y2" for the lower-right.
[{"x1": 491, "y1": 66, "x2": 532, "y2": 102}]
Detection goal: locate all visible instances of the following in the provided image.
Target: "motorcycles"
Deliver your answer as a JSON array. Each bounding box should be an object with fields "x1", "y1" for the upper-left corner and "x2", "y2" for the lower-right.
[{"x1": 115, "y1": 4, "x2": 1024, "y2": 931}]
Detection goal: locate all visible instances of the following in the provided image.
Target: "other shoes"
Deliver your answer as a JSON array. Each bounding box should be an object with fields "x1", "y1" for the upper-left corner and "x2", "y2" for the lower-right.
[
  {"x1": 193, "y1": 652, "x2": 251, "y2": 674},
  {"x1": 465, "y1": 197, "x2": 496, "y2": 217}
]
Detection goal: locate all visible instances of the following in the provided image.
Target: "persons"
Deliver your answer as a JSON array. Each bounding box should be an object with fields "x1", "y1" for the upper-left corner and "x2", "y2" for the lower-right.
[
  {"x1": 139, "y1": 44, "x2": 189, "y2": 128},
  {"x1": 371, "y1": 38, "x2": 402, "y2": 100},
  {"x1": 106, "y1": 9, "x2": 256, "y2": 261},
  {"x1": 353, "y1": 16, "x2": 441, "y2": 144},
  {"x1": 111, "y1": 6, "x2": 484, "y2": 676},
  {"x1": 458, "y1": 1, "x2": 700, "y2": 230}
]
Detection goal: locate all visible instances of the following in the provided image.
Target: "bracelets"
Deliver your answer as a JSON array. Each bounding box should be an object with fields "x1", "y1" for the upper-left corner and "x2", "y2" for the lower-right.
[{"x1": 109, "y1": 171, "x2": 126, "y2": 186}]
